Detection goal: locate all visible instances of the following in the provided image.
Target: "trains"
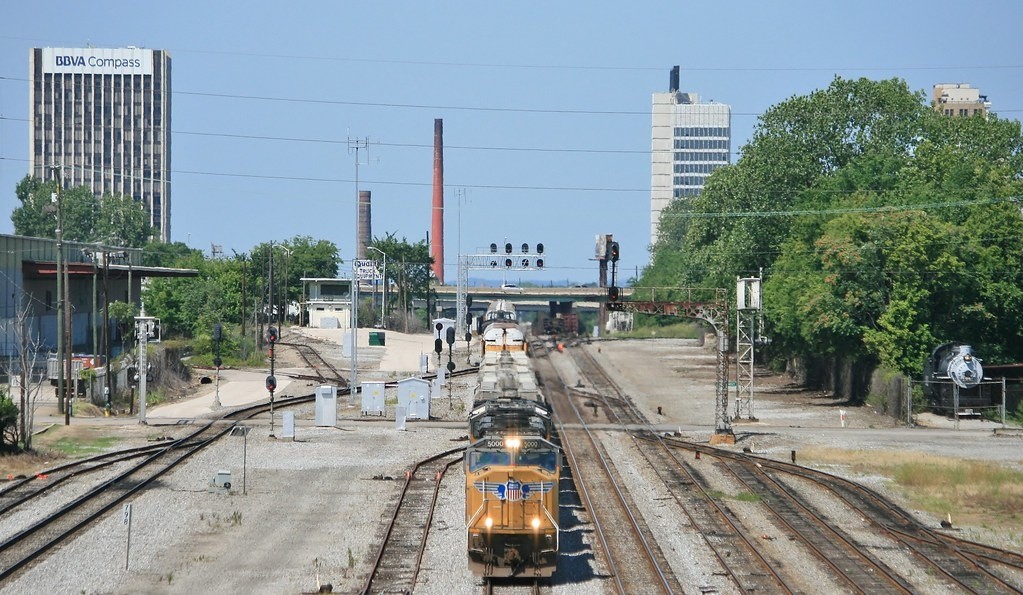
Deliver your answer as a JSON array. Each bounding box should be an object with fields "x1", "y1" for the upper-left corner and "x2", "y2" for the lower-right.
[
  {"x1": 462, "y1": 295, "x2": 561, "y2": 581},
  {"x1": 558, "y1": 314, "x2": 578, "y2": 333}
]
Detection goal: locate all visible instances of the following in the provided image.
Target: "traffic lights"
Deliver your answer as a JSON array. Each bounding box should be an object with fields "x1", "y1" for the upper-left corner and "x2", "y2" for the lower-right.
[
  {"x1": 608, "y1": 286, "x2": 619, "y2": 301},
  {"x1": 268, "y1": 326, "x2": 277, "y2": 344},
  {"x1": 214, "y1": 324, "x2": 221, "y2": 342},
  {"x1": 265, "y1": 375, "x2": 276, "y2": 390},
  {"x1": 610, "y1": 242, "x2": 619, "y2": 263},
  {"x1": 537, "y1": 259, "x2": 543, "y2": 267},
  {"x1": 506, "y1": 259, "x2": 512, "y2": 267}
]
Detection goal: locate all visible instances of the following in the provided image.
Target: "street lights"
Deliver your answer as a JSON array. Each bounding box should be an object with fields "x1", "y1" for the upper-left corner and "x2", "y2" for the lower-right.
[{"x1": 367, "y1": 245, "x2": 387, "y2": 328}]
